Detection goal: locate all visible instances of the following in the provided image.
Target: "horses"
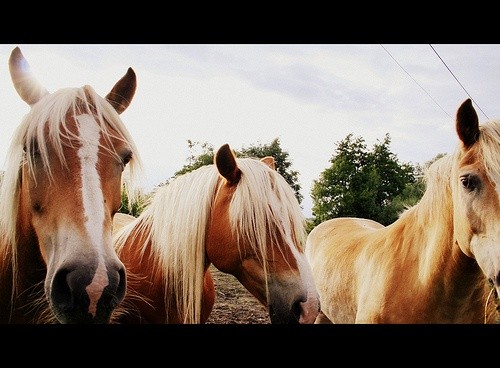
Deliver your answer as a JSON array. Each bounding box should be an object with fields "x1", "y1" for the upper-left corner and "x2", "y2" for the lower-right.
[
  {"x1": 112, "y1": 143, "x2": 321, "y2": 325},
  {"x1": 304, "y1": 98, "x2": 500, "y2": 324},
  {"x1": 0, "y1": 46, "x2": 155, "y2": 325}
]
